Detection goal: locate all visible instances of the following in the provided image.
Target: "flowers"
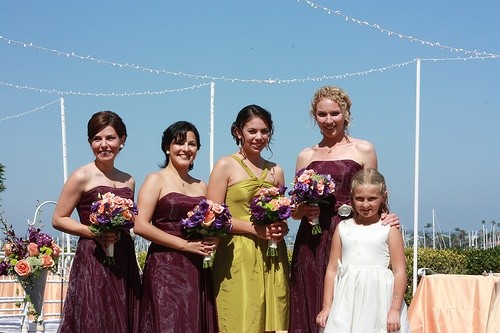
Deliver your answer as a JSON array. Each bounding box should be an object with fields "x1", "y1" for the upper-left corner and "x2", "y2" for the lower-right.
[
  {"x1": 288, "y1": 170, "x2": 336, "y2": 235},
  {"x1": 87, "y1": 192, "x2": 139, "y2": 265},
  {"x1": 0, "y1": 209, "x2": 62, "y2": 333},
  {"x1": 179, "y1": 198, "x2": 233, "y2": 269},
  {"x1": 336, "y1": 258, "x2": 344, "y2": 276},
  {"x1": 248, "y1": 183, "x2": 291, "y2": 256},
  {"x1": 338, "y1": 204, "x2": 353, "y2": 217}
]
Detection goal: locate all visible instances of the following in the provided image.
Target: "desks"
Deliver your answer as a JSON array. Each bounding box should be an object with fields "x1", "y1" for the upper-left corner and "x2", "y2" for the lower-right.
[{"x1": 408, "y1": 273, "x2": 500, "y2": 333}]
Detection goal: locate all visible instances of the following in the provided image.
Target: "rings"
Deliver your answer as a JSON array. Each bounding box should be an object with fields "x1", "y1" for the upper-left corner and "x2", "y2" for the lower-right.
[{"x1": 102, "y1": 240, "x2": 106, "y2": 243}]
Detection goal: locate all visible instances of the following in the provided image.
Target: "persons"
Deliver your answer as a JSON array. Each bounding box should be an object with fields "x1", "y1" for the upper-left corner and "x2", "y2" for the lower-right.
[
  {"x1": 206, "y1": 104, "x2": 292, "y2": 333},
  {"x1": 289, "y1": 86, "x2": 402, "y2": 333},
  {"x1": 52, "y1": 111, "x2": 142, "y2": 333},
  {"x1": 134, "y1": 121, "x2": 220, "y2": 333},
  {"x1": 316, "y1": 168, "x2": 409, "y2": 333}
]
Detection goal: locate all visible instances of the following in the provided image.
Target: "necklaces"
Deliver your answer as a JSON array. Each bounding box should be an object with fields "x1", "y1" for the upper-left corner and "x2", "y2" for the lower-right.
[{"x1": 174, "y1": 174, "x2": 184, "y2": 187}]
